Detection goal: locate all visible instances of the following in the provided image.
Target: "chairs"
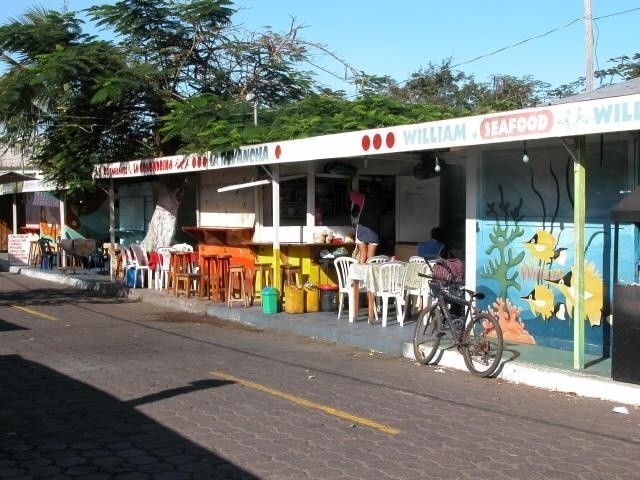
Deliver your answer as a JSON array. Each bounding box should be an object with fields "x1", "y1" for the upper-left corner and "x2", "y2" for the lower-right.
[
  {"x1": 333, "y1": 254, "x2": 464, "y2": 329},
  {"x1": 38, "y1": 236, "x2": 193, "y2": 293}
]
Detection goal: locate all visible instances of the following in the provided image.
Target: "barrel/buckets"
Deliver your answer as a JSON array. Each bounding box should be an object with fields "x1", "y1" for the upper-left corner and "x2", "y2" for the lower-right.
[{"x1": 261, "y1": 287, "x2": 281, "y2": 315}]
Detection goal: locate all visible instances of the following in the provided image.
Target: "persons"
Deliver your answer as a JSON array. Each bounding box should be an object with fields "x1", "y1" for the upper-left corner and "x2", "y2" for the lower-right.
[
  {"x1": 416, "y1": 226, "x2": 462, "y2": 317},
  {"x1": 350, "y1": 180, "x2": 382, "y2": 264}
]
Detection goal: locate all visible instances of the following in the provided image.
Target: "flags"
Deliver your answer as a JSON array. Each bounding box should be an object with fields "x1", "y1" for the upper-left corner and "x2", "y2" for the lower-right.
[{"x1": 348, "y1": 190, "x2": 365, "y2": 208}]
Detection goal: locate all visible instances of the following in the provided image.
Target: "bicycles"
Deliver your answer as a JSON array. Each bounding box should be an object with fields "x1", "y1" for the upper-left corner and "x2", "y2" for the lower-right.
[{"x1": 413, "y1": 272, "x2": 504, "y2": 378}]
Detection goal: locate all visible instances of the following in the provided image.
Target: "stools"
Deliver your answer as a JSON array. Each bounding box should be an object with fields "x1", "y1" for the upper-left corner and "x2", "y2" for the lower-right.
[
  {"x1": 170, "y1": 250, "x2": 302, "y2": 308},
  {"x1": 28, "y1": 241, "x2": 41, "y2": 268}
]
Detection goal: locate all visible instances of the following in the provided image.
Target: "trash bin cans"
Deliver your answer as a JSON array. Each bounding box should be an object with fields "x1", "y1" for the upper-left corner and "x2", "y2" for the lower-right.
[
  {"x1": 261, "y1": 284, "x2": 339, "y2": 314},
  {"x1": 127, "y1": 268, "x2": 142, "y2": 288}
]
